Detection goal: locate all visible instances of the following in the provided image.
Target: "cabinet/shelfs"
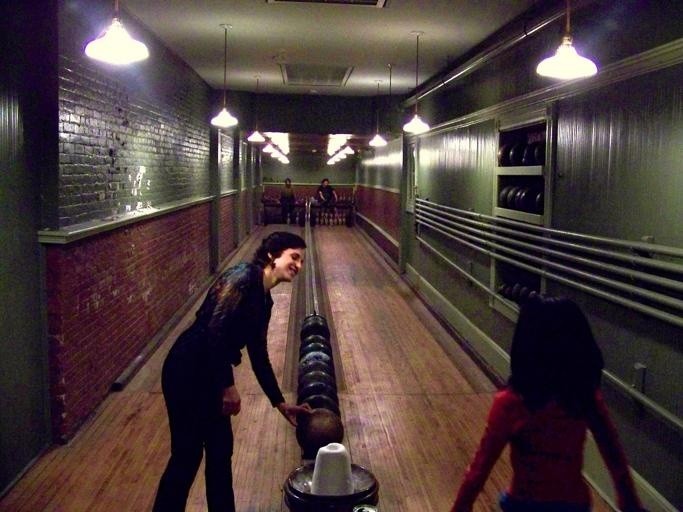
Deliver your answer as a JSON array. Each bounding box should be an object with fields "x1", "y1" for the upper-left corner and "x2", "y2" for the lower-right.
[{"x1": 487, "y1": 101, "x2": 555, "y2": 323}]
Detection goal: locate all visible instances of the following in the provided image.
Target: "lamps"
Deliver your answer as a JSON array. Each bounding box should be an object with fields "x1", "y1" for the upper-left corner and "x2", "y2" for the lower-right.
[
  {"x1": 209, "y1": 23, "x2": 267, "y2": 143},
  {"x1": 367, "y1": 32, "x2": 429, "y2": 147},
  {"x1": 536, "y1": 0, "x2": 597, "y2": 81},
  {"x1": 84, "y1": 1, "x2": 148, "y2": 65}
]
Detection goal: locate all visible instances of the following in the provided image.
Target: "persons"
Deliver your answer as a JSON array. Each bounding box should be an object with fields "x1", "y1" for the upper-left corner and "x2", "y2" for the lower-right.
[
  {"x1": 279, "y1": 177, "x2": 296, "y2": 220},
  {"x1": 447, "y1": 292, "x2": 647, "y2": 511},
  {"x1": 149, "y1": 231, "x2": 312, "y2": 511},
  {"x1": 314, "y1": 178, "x2": 338, "y2": 212}
]
